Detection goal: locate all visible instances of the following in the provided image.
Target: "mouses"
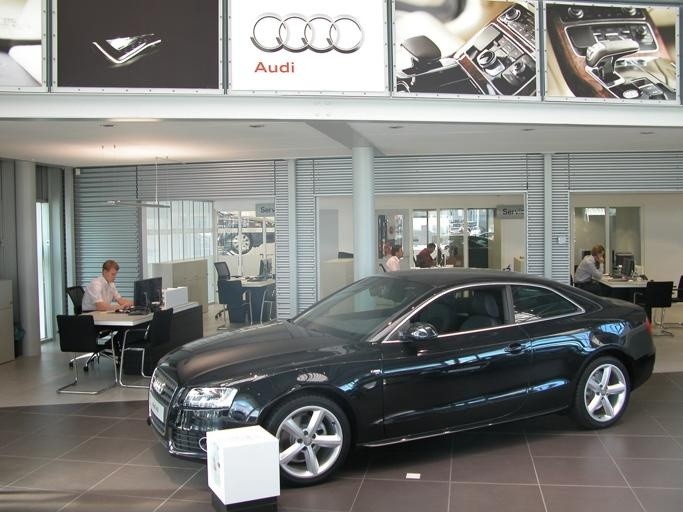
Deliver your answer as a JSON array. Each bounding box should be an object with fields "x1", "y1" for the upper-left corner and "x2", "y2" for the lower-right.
[{"x1": 115, "y1": 309, "x2": 124, "y2": 313}]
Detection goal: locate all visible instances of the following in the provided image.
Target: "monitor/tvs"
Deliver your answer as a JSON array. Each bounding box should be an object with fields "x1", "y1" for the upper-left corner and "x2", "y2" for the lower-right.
[
  {"x1": 259, "y1": 257, "x2": 272, "y2": 275},
  {"x1": 214, "y1": 262, "x2": 230, "y2": 277},
  {"x1": 622, "y1": 259, "x2": 634, "y2": 277},
  {"x1": 437, "y1": 247, "x2": 442, "y2": 264},
  {"x1": 133, "y1": 276, "x2": 163, "y2": 306}
]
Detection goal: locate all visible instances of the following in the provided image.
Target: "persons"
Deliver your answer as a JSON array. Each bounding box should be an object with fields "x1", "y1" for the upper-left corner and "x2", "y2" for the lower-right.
[
  {"x1": 82, "y1": 259, "x2": 134, "y2": 367},
  {"x1": 385, "y1": 244, "x2": 404, "y2": 271},
  {"x1": 417, "y1": 242, "x2": 436, "y2": 268},
  {"x1": 573, "y1": 244, "x2": 605, "y2": 294}
]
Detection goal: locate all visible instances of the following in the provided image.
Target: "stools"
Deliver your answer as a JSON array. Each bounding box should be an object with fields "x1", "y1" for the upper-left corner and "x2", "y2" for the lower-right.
[{"x1": 205, "y1": 424, "x2": 281, "y2": 511}]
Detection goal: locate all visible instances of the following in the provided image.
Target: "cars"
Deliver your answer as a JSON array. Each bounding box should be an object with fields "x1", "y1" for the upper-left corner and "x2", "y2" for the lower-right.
[{"x1": 149, "y1": 269, "x2": 654, "y2": 486}]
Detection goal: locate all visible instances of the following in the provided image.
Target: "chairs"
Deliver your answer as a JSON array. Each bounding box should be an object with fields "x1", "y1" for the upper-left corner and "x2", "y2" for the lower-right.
[
  {"x1": 259, "y1": 283, "x2": 277, "y2": 326},
  {"x1": 633, "y1": 281, "x2": 673, "y2": 337},
  {"x1": 118, "y1": 308, "x2": 174, "y2": 390},
  {"x1": 213, "y1": 261, "x2": 244, "y2": 320},
  {"x1": 54, "y1": 314, "x2": 117, "y2": 395},
  {"x1": 654, "y1": 274, "x2": 683, "y2": 329},
  {"x1": 217, "y1": 280, "x2": 251, "y2": 330},
  {"x1": 66, "y1": 286, "x2": 121, "y2": 372}
]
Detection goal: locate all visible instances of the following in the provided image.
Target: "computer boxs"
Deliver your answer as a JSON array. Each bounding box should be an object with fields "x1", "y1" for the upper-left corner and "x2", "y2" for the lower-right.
[{"x1": 616, "y1": 254, "x2": 632, "y2": 266}]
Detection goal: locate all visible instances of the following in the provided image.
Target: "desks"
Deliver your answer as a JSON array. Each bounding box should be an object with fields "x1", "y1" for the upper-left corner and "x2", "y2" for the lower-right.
[
  {"x1": 591, "y1": 273, "x2": 649, "y2": 302},
  {"x1": 234, "y1": 277, "x2": 276, "y2": 325},
  {"x1": 79, "y1": 300, "x2": 204, "y2": 379}
]
